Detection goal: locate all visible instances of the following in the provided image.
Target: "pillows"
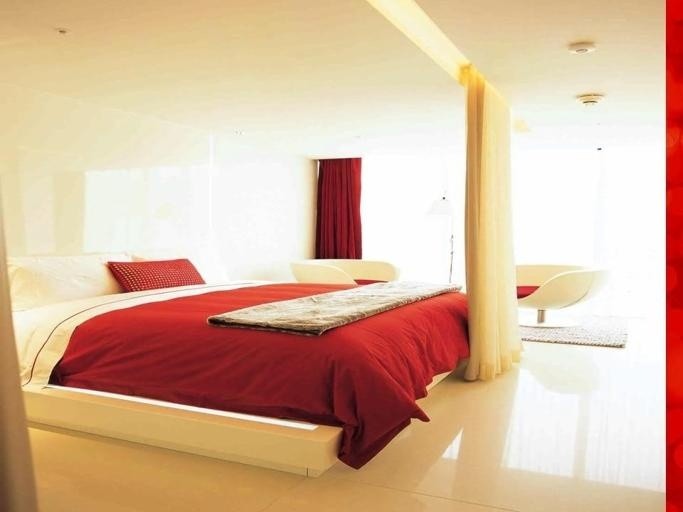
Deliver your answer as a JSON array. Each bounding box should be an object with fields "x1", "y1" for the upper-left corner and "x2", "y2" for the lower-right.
[
  {"x1": 7, "y1": 256, "x2": 120, "y2": 310},
  {"x1": 109, "y1": 257, "x2": 207, "y2": 295}
]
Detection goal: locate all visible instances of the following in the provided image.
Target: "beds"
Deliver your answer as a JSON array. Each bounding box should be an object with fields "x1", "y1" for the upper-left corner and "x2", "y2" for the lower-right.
[{"x1": 1, "y1": 280, "x2": 471, "y2": 482}]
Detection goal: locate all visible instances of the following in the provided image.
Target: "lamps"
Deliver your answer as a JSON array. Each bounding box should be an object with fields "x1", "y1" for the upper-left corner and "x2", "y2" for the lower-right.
[{"x1": 571, "y1": 41, "x2": 593, "y2": 56}]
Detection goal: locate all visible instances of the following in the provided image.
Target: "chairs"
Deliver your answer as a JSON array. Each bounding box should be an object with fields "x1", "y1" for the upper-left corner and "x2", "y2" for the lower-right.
[
  {"x1": 292, "y1": 256, "x2": 396, "y2": 286},
  {"x1": 513, "y1": 263, "x2": 598, "y2": 330}
]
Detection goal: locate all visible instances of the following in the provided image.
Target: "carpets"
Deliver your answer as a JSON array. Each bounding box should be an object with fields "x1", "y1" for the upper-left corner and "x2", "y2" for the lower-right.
[{"x1": 515, "y1": 325, "x2": 628, "y2": 348}]
